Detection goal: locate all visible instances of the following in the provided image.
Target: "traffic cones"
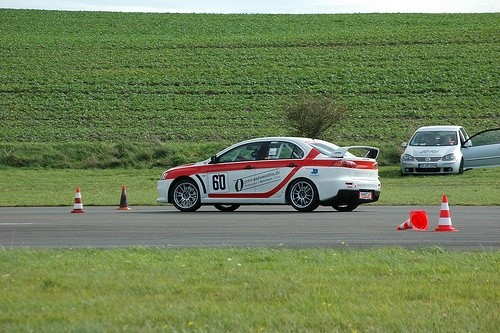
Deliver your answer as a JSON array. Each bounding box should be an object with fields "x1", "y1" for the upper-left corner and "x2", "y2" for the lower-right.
[
  {"x1": 396, "y1": 210, "x2": 428, "y2": 232},
  {"x1": 115, "y1": 185, "x2": 131, "y2": 210},
  {"x1": 434, "y1": 195, "x2": 459, "y2": 232},
  {"x1": 70, "y1": 187, "x2": 86, "y2": 213}
]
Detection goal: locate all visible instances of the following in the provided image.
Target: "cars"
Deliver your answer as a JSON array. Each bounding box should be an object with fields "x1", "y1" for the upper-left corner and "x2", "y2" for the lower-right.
[
  {"x1": 399, "y1": 125, "x2": 500, "y2": 175},
  {"x1": 155, "y1": 137, "x2": 381, "y2": 212}
]
